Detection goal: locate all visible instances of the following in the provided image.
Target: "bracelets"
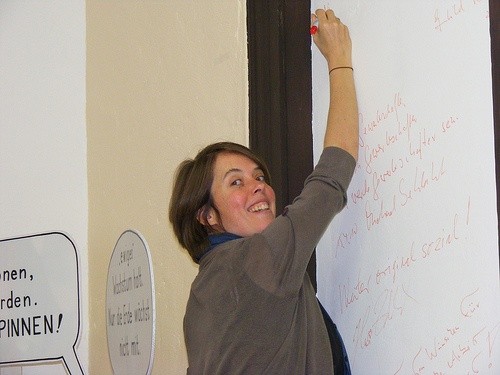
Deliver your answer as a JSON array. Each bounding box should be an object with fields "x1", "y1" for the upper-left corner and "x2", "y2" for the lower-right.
[{"x1": 329, "y1": 66, "x2": 354, "y2": 75}]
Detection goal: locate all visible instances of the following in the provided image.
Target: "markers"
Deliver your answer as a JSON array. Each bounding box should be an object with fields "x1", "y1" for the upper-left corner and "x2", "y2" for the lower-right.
[{"x1": 309, "y1": 22, "x2": 318, "y2": 36}]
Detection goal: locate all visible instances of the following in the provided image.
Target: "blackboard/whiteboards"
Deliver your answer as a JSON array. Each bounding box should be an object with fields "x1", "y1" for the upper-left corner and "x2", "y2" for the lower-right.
[{"x1": 310, "y1": 0, "x2": 500, "y2": 375}]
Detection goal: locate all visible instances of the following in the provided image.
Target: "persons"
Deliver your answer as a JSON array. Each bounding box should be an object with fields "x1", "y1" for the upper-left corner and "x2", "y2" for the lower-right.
[{"x1": 168, "y1": 9, "x2": 360, "y2": 375}]
